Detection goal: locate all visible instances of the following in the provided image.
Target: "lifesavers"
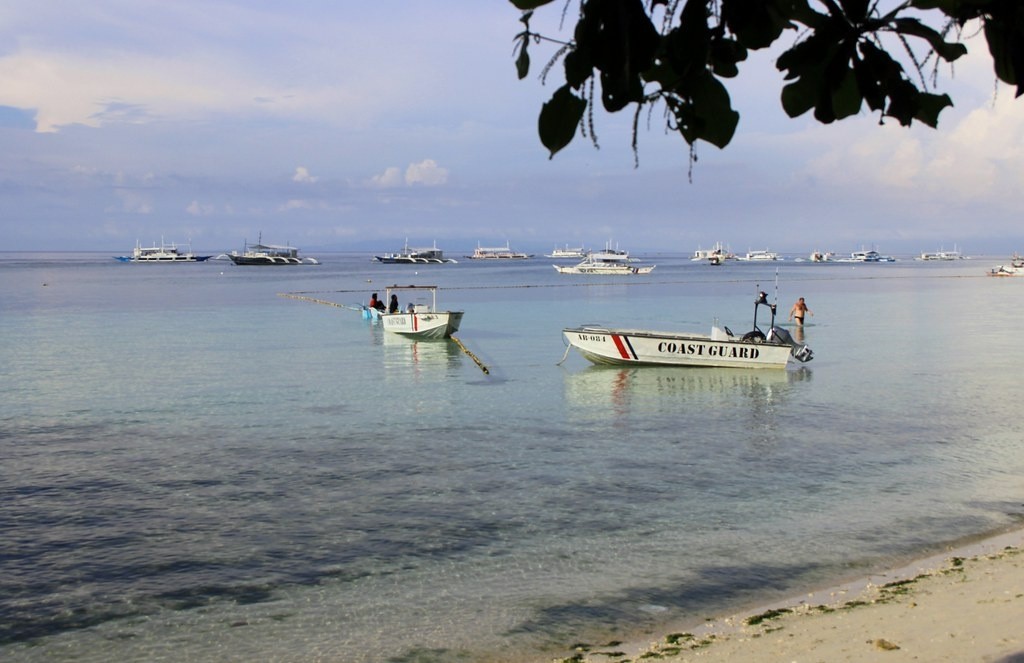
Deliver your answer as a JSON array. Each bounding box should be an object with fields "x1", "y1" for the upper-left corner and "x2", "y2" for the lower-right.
[{"x1": 743, "y1": 331, "x2": 766, "y2": 343}]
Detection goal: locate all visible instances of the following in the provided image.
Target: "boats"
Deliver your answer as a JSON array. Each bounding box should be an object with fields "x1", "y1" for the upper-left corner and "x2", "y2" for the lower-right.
[
  {"x1": 111, "y1": 235, "x2": 215, "y2": 264},
  {"x1": 214, "y1": 231, "x2": 323, "y2": 266},
  {"x1": 359, "y1": 284, "x2": 464, "y2": 337},
  {"x1": 544, "y1": 239, "x2": 657, "y2": 276},
  {"x1": 691, "y1": 238, "x2": 1024, "y2": 277},
  {"x1": 561, "y1": 267, "x2": 814, "y2": 370},
  {"x1": 374, "y1": 238, "x2": 535, "y2": 264}
]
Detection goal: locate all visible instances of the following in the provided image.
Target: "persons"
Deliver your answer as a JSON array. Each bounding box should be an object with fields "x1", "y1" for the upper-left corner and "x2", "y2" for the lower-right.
[
  {"x1": 369, "y1": 293, "x2": 387, "y2": 310},
  {"x1": 406, "y1": 303, "x2": 414, "y2": 314},
  {"x1": 788, "y1": 297, "x2": 813, "y2": 326},
  {"x1": 999, "y1": 265, "x2": 1005, "y2": 272},
  {"x1": 390, "y1": 295, "x2": 400, "y2": 313}
]
